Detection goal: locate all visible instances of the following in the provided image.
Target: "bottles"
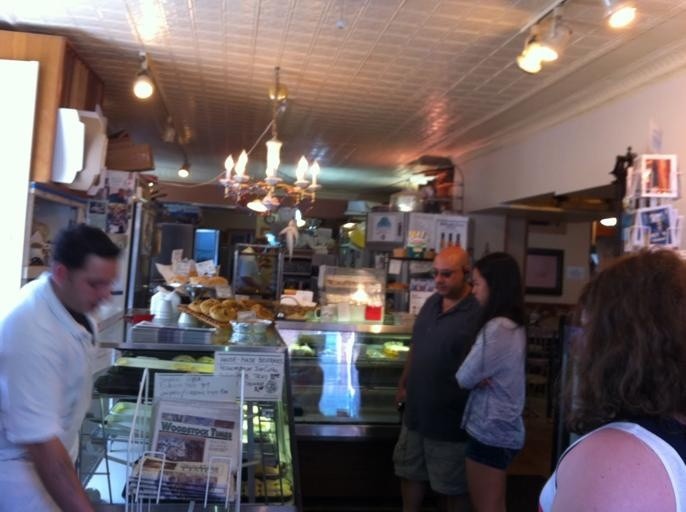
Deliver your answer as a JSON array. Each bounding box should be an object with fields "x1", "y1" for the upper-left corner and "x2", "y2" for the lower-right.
[
  {"x1": 427, "y1": 248, "x2": 437, "y2": 259},
  {"x1": 439, "y1": 232, "x2": 460, "y2": 250}
]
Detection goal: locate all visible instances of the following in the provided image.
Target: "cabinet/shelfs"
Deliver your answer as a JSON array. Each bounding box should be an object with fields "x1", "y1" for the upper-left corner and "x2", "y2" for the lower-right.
[
  {"x1": 20, "y1": 175, "x2": 88, "y2": 298},
  {"x1": 85, "y1": 304, "x2": 301, "y2": 511},
  {"x1": 225, "y1": 226, "x2": 478, "y2": 316},
  {"x1": 0, "y1": 27, "x2": 111, "y2": 204},
  {"x1": 271, "y1": 306, "x2": 426, "y2": 446}
]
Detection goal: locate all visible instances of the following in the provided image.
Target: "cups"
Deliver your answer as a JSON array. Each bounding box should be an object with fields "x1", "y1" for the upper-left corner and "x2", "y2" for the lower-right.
[
  {"x1": 147, "y1": 289, "x2": 198, "y2": 326},
  {"x1": 314, "y1": 305, "x2": 338, "y2": 321}
]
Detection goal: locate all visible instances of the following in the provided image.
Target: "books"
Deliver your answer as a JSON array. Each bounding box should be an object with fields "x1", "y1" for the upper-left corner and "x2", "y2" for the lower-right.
[
  {"x1": 622, "y1": 153, "x2": 683, "y2": 251},
  {"x1": 129, "y1": 397, "x2": 240, "y2": 504}
]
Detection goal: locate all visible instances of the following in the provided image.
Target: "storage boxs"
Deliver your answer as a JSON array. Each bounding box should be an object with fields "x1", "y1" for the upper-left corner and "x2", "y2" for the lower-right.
[{"x1": 109, "y1": 142, "x2": 155, "y2": 172}]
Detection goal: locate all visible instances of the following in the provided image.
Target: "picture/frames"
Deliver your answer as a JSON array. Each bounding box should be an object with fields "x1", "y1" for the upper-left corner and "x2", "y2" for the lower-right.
[{"x1": 525, "y1": 246, "x2": 564, "y2": 297}]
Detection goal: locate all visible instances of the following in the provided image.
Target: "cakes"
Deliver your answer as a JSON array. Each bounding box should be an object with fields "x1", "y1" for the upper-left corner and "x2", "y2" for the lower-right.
[
  {"x1": 382, "y1": 340, "x2": 408, "y2": 358},
  {"x1": 318, "y1": 273, "x2": 386, "y2": 306}
]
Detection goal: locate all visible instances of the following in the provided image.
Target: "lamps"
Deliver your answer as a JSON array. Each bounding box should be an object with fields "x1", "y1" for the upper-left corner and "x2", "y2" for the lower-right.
[
  {"x1": 129, "y1": 44, "x2": 194, "y2": 180},
  {"x1": 214, "y1": 66, "x2": 323, "y2": 224},
  {"x1": 598, "y1": 145, "x2": 640, "y2": 229},
  {"x1": 513, "y1": 0, "x2": 638, "y2": 75}
]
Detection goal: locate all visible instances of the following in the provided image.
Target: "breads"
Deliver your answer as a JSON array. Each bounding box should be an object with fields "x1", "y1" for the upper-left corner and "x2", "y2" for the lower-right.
[
  {"x1": 169, "y1": 273, "x2": 227, "y2": 289},
  {"x1": 239, "y1": 477, "x2": 293, "y2": 497},
  {"x1": 189, "y1": 295, "x2": 278, "y2": 324},
  {"x1": 173, "y1": 354, "x2": 214, "y2": 364}
]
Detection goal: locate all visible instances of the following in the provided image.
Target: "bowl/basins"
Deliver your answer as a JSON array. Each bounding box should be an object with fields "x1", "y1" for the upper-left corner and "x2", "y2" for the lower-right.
[{"x1": 230, "y1": 320, "x2": 273, "y2": 335}]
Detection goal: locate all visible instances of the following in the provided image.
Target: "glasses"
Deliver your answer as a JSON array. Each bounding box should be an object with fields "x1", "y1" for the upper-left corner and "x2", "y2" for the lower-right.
[{"x1": 429, "y1": 266, "x2": 465, "y2": 278}]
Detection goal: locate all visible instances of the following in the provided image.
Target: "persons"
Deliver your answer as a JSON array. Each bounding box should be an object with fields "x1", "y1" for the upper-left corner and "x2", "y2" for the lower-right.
[
  {"x1": 393, "y1": 245, "x2": 481, "y2": 512},
  {"x1": 536, "y1": 246, "x2": 686, "y2": 512},
  {"x1": 0, "y1": 220, "x2": 121, "y2": 512},
  {"x1": 455, "y1": 250, "x2": 526, "y2": 512}
]
